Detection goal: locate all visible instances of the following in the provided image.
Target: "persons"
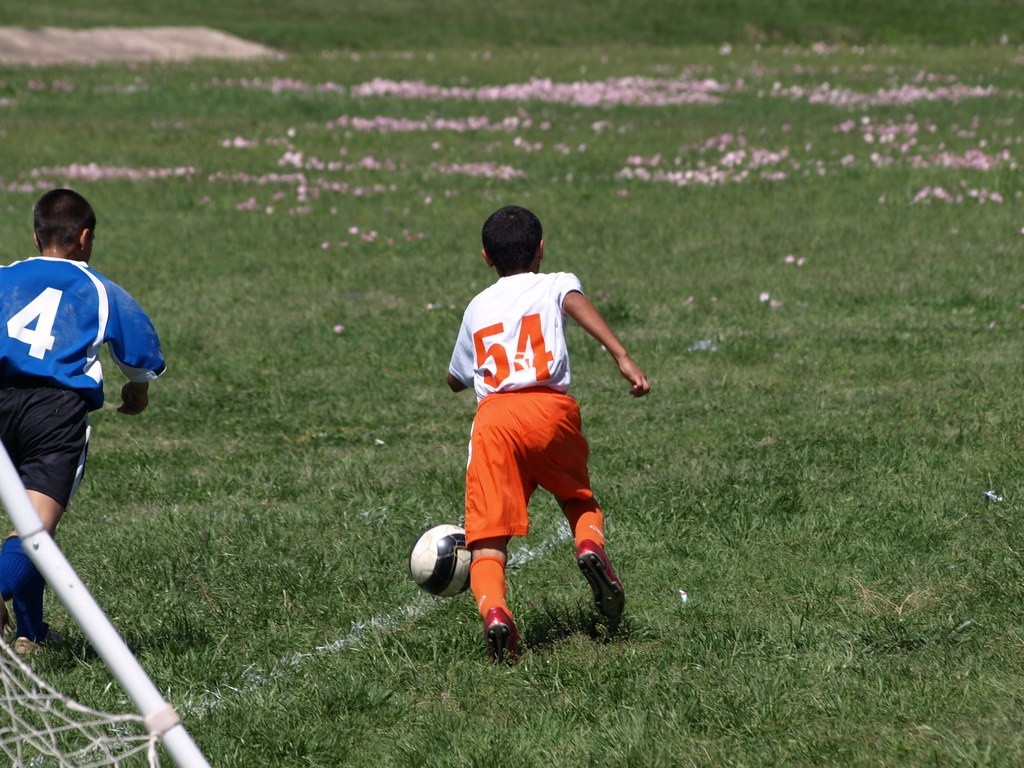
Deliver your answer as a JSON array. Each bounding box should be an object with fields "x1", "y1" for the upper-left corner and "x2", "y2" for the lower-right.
[
  {"x1": 0, "y1": 188, "x2": 167, "y2": 655},
  {"x1": 445, "y1": 203, "x2": 652, "y2": 670}
]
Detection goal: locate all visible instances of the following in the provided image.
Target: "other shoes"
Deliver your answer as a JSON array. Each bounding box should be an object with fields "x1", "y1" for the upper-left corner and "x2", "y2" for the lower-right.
[
  {"x1": 0, "y1": 594, "x2": 17, "y2": 638},
  {"x1": 14, "y1": 632, "x2": 64, "y2": 654},
  {"x1": 576, "y1": 539, "x2": 625, "y2": 618},
  {"x1": 484, "y1": 607, "x2": 519, "y2": 667}
]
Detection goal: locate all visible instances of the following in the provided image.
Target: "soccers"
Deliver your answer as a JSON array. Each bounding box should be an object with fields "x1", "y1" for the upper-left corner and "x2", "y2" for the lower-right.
[{"x1": 408, "y1": 524, "x2": 472, "y2": 597}]
